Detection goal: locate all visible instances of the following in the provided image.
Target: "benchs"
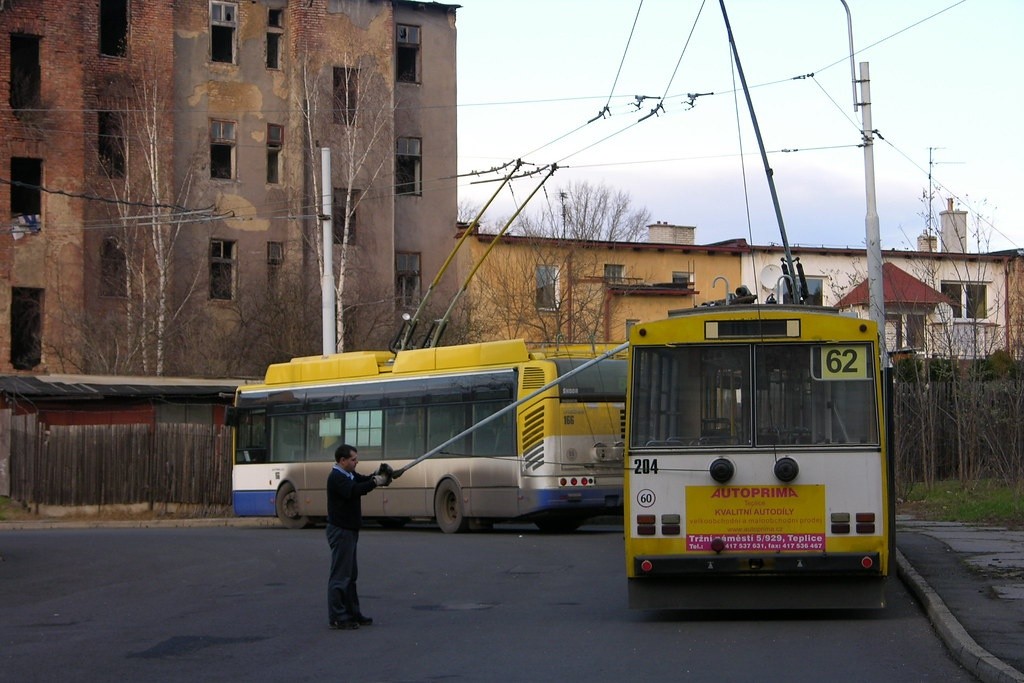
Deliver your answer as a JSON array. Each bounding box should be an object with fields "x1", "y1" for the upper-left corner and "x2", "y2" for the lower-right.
[{"x1": 406, "y1": 430, "x2": 513, "y2": 456}]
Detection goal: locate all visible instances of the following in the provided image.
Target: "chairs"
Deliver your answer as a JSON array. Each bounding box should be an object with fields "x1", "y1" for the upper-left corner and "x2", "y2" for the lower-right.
[{"x1": 646, "y1": 426, "x2": 826, "y2": 446}]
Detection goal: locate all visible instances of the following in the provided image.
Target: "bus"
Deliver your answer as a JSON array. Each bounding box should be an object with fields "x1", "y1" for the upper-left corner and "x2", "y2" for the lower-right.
[
  {"x1": 379, "y1": 0, "x2": 891, "y2": 611},
  {"x1": 222, "y1": 159, "x2": 630, "y2": 536}
]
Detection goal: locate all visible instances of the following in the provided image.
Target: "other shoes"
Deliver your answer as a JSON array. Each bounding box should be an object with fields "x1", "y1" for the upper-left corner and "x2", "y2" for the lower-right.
[
  {"x1": 328, "y1": 617, "x2": 359, "y2": 629},
  {"x1": 347, "y1": 612, "x2": 373, "y2": 625}
]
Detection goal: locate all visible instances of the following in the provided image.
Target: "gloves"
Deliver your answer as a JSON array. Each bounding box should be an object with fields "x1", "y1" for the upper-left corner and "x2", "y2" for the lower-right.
[{"x1": 371, "y1": 462, "x2": 394, "y2": 486}]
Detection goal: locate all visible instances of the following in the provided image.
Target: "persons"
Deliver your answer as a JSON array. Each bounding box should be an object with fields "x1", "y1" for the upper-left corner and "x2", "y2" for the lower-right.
[{"x1": 326, "y1": 445, "x2": 393, "y2": 630}]
